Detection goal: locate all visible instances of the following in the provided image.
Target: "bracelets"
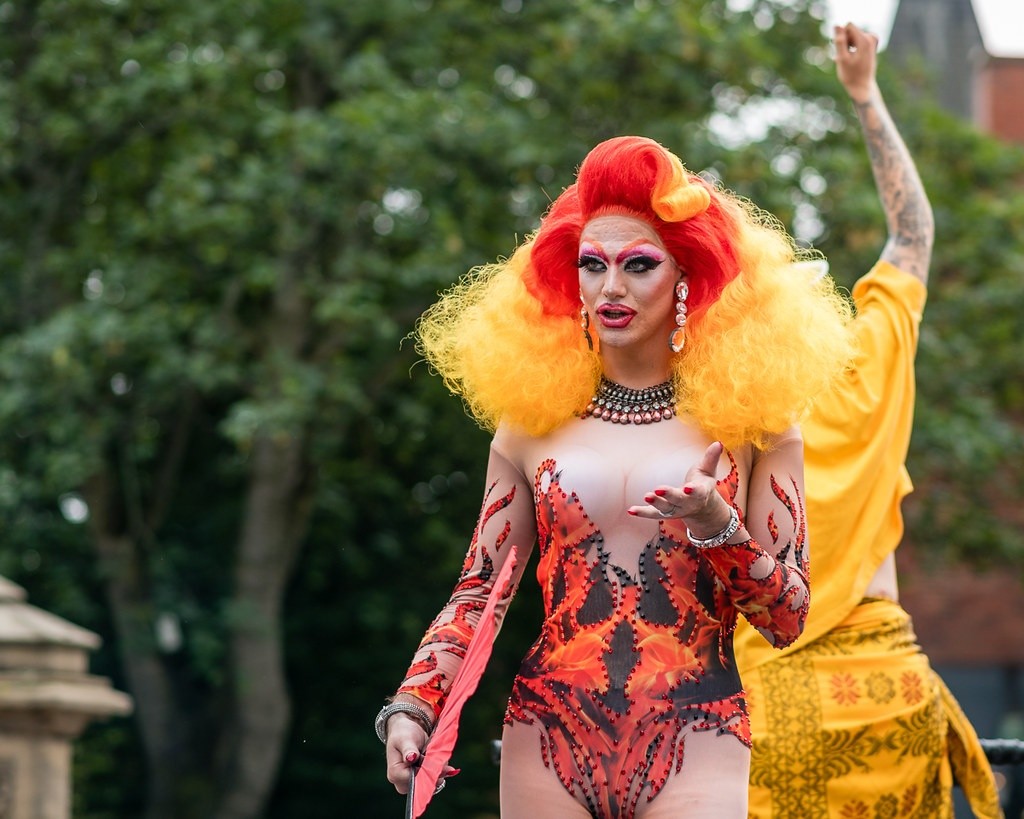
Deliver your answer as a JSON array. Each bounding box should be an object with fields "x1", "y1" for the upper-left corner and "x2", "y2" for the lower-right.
[
  {"x1": 685, "y1": 505, "x2": 739, "y2": 549},
  {"x1": 374, "y1": 701, "x2": 433, "y2": 744}
]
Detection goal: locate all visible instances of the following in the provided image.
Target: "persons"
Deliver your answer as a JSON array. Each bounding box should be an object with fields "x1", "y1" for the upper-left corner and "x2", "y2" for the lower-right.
[
  {"x1": 370, "y1": 134, "x2": 816, "y2": 818},
  {"x1": 732, "y1": 20, "x2": 1006, "y2": 819}
]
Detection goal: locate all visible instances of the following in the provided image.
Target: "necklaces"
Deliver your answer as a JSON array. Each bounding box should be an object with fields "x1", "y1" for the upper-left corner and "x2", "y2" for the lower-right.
[{"x1": 574, "y1": 372, "x2": 686, "y2": 426}]
